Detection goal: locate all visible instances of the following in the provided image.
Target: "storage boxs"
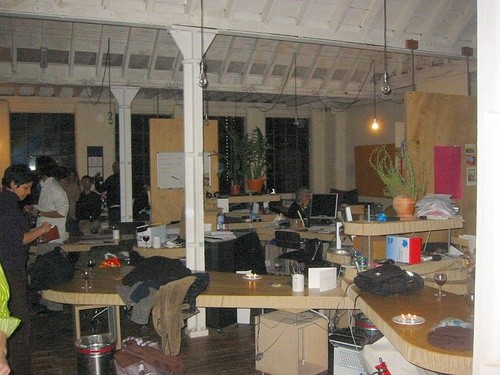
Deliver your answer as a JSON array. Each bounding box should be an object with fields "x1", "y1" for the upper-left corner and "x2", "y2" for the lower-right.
[{"x1": 386, "y1": 235, "x2": 423, "y2": 264}]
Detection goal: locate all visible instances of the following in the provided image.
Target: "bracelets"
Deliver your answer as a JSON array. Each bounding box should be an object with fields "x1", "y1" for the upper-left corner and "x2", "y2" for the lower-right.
[{"x1": 37, "y1": 211, "x2": 40, "y2": 217}]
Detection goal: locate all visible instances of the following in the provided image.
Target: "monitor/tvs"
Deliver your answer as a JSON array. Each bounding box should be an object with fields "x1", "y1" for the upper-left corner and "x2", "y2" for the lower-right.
[{"x1": 309, "y1": 192, "x2": 338, "y2": 224}]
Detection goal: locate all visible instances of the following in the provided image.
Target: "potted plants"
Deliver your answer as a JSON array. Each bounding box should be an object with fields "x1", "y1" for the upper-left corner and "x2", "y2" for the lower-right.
[
  {"x1": 208, "y1": 125, "x2": 269, "y2": 195},
  {"x1": 368, "y1": 142, "x2": 428, "y2": 218}
]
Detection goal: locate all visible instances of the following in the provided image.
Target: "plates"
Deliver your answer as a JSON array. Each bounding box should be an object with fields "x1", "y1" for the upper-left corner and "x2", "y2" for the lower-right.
[
  {"x1": 242, "y1": 275, "x2": 262, "y2": 280},
  {"x1": 392, "y1": 315, "x2": 425, "y2": 325}
]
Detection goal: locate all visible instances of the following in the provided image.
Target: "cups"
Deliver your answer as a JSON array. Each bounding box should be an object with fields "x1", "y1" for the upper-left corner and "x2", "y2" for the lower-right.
[
  {"x1": 152, "y1": 237, "x2": 162, "y2": 248},
  {"x1": 292, "y1": 274, "x2": 304, "y2": 292},
  {"x1": 112, "y1": 230, "x2": 120, "y2": 240}
]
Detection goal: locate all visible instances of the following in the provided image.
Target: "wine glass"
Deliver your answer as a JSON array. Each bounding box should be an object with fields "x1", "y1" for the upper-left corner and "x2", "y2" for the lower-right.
[
  {"x1": 143, "y1": 235, "x2": 150, "y2": 249},
  {"x1": 434, "y1": 272, "x2": 448, "y2": 296},
  {"x1": 465, "y1": 292, "x2": 474, "y2": 320},
  {"x1": 82, "y1": 257, "x2": 96, "y2": 289}
]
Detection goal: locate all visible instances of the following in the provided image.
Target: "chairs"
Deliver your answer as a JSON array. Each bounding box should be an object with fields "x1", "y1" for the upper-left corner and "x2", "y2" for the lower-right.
[{"x1": 274, "y1": 230, "x2": 307, "y2": 275}]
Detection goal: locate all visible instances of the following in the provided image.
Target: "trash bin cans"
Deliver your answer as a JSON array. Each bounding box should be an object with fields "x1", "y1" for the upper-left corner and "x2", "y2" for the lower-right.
[{"x1": 74, "y1": 334, "x2": 117, "y2": 375}]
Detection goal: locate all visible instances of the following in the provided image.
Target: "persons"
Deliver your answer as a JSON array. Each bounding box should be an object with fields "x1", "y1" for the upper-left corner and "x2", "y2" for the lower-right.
[
  {"x1": 0, "y1": 155, "x2": 80, "y2": 375},
  {"x1": 94, "y1": 161, "x2": 120, "y2": 222},
  {"x1": 288, "y1": 186, "x2": 311, "y2": 219},
  {"x1": 75, "y1": 175, "x2": 102, "y2": 221}
]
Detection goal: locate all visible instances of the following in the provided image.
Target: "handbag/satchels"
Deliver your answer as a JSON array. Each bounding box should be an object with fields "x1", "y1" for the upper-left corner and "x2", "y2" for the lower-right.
[
  {"x1": 354, "y1": 258, "x2": 425, "y2": 297},
  {"x1": 27, "y1": 246, "x2": 75, "y2": 293}
]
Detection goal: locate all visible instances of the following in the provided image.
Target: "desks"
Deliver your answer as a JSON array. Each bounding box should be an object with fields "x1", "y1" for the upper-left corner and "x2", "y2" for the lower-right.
[{"x1": 29, "y1": 214, "x2": 473, "y2": 375}]
